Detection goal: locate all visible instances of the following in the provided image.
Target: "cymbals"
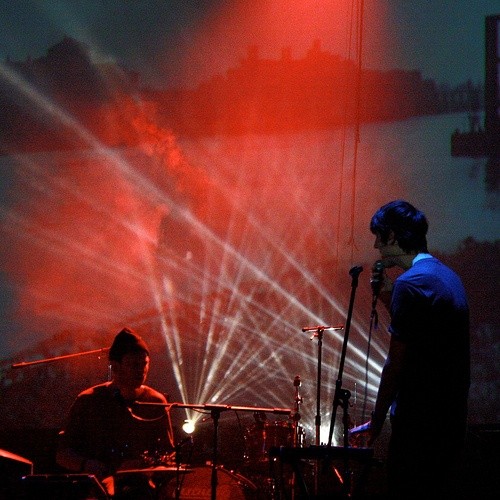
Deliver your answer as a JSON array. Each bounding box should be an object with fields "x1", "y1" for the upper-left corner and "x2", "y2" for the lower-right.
[{"x1": 118, "y1": 467, "x2": 192, "y2": 477}]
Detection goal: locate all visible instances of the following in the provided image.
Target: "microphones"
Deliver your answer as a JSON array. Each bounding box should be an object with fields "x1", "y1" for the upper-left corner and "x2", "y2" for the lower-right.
[
  {"x1": 372, "y1": 260, "x2": 385, "y2": 295},
  {"x1": 294, "y1": 376, "x2": 302, "y2": 387},
  {"x1": 166, "y1": 435, "x2": 193, "y2": 459}
]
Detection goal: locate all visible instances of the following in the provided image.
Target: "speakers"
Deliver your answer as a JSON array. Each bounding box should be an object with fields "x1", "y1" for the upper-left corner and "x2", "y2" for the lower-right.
[{"x1": 0, "y1": 448, "x2": 108, "y2": 500}]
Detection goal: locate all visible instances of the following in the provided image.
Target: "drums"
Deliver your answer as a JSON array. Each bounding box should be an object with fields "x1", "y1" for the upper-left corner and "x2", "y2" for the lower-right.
[
  {"x1": 163, "y1": 467, "x2": 245, "y2": 500},
  {"x1": 243, "y1": 421, "x2": 305, "y2": 464}
]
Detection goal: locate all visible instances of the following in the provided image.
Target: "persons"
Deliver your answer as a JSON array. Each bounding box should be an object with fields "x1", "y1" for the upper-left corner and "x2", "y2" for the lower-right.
[
  {"x1": 349, "y1": 200, "x2": 472, "y2": 499},
  {"x1": 55, "y1": 327, "x2": 176, "y2": 500}
]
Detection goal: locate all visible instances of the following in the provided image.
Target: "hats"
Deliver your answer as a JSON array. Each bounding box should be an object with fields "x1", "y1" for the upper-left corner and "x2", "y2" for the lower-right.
[{"x1": 108, "y1": 327, "x2": 150, "y2": 360}]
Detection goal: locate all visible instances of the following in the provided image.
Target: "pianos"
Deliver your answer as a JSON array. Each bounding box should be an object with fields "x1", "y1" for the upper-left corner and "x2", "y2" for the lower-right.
[{"x1": 270, "y1": 446, "x2": 374, "y2": 463}]
